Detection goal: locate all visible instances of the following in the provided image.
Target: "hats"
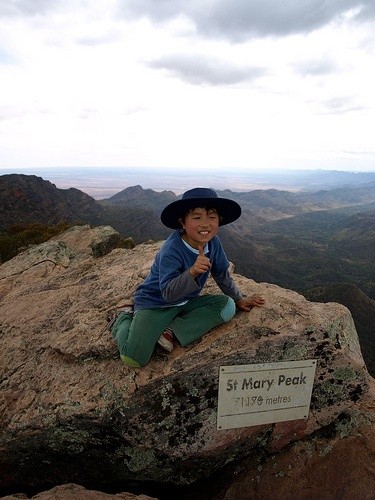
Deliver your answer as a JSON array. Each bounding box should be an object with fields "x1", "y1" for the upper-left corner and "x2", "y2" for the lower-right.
[{"x1": 161, "y1": 188, "x2": 242, "y2": 229}]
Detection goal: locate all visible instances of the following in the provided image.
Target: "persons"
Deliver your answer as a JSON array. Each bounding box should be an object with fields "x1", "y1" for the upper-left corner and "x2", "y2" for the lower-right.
[{"x1": 104, "y1": 188, "x2": 266, "y2": 368}]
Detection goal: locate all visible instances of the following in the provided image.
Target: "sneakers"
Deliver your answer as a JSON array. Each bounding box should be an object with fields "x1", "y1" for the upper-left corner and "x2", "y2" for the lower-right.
[
  {"x1": 105, "y1": 304, "x2": 133, "y2": 323},
  {"x1": 159, "y1": 328, "x2": 174, "y2": 352}
]
